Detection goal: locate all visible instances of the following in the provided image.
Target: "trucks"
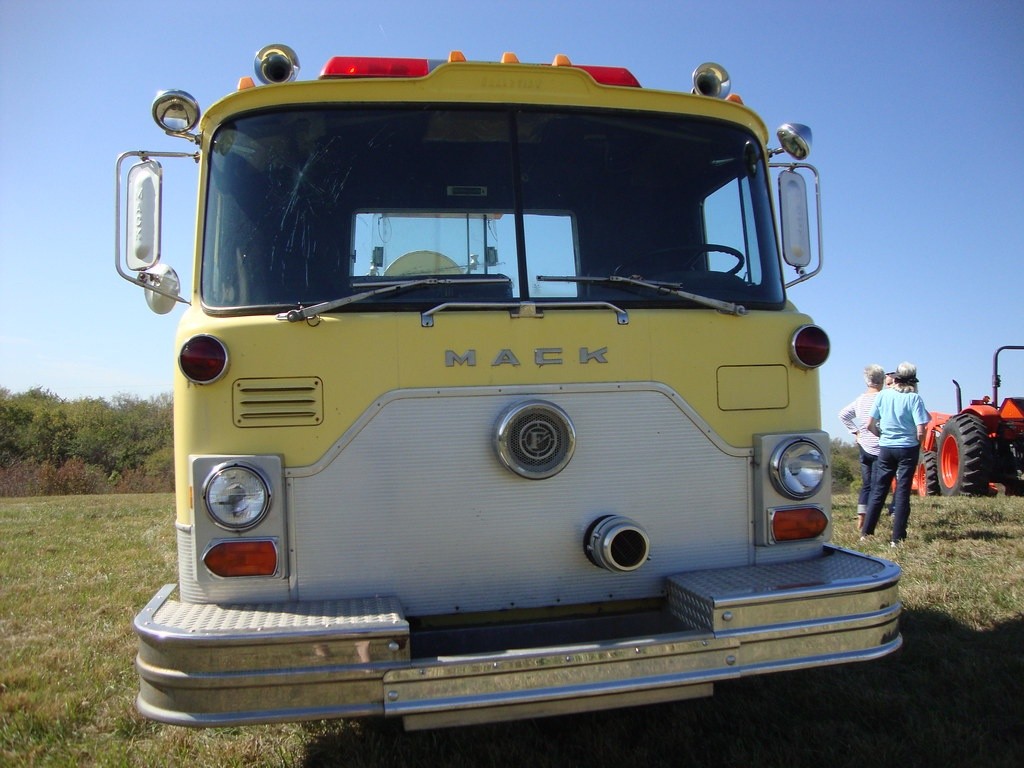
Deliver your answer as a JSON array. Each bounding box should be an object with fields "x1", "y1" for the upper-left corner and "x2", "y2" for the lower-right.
[{"x1": 117, "y1": 47, "x2": 903, "y2": 730}]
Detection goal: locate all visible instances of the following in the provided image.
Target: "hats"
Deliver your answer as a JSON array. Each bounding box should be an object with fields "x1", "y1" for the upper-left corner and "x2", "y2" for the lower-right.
[{"x1": 886, "y1": 362, "x2": 919, "y2": 382}]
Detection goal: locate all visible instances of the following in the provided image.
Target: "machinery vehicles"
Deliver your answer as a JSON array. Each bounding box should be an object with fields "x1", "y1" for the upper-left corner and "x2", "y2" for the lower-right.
[{"x1": 891, "y1": 343, "x2": 1024, "y2": 498}]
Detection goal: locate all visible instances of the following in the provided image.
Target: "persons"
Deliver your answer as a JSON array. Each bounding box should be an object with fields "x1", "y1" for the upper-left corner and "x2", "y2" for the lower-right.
[
  {"x1": 839, "y1": 364, "x2": 914, "y2": 532},
  {"x1": 860, "y1": 361, "x2": 931, "y2": 547}
]
